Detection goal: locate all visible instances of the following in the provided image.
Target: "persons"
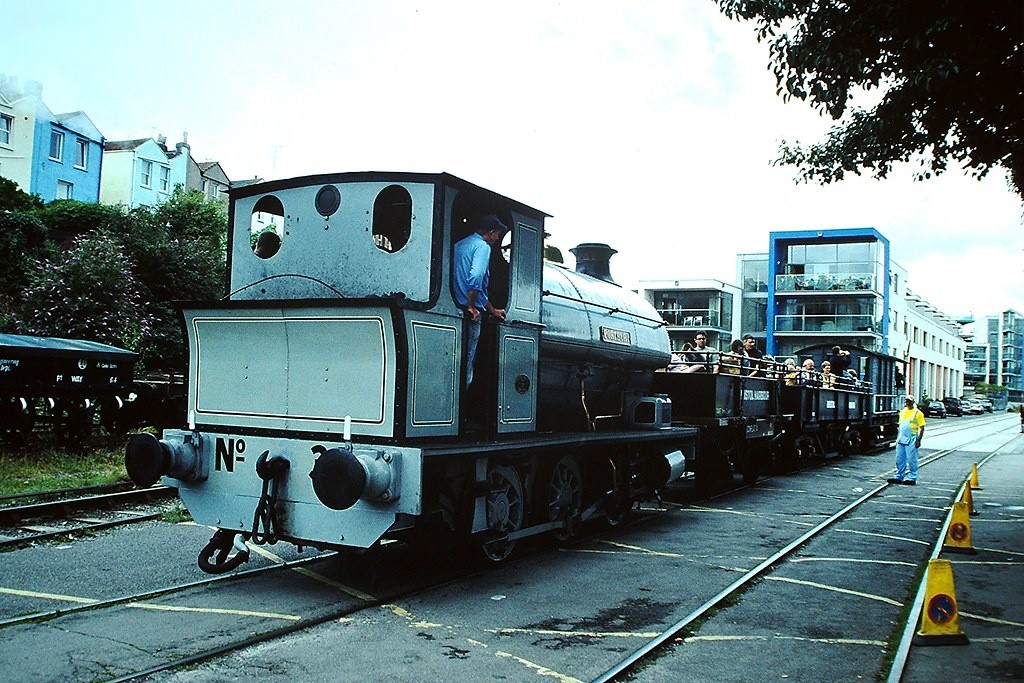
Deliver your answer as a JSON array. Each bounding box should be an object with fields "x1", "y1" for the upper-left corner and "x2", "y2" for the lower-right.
[
  {"x1": 667, "y1": 331, "x2": 857, "y2": 389},
  {"x1": 451, "y1": 216, "x2": 508, "y2": 410},
  {"x1": 888, "y1": 396, "x2": 927, "y2": 486}
]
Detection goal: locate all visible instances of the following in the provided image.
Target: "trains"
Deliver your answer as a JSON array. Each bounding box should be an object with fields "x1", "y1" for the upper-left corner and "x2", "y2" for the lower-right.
[{"x1": 124, "y1": 169, "x2": 911, "y2": 572}]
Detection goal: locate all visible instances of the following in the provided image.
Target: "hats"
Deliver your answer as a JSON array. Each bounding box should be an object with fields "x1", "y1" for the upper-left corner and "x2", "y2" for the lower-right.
[
  {"x1": 482, "y1": 215, "x2": 508, "y2": 231},
  {"x1": 905, "y1": 395, "x2": 914, "y2": 402}
]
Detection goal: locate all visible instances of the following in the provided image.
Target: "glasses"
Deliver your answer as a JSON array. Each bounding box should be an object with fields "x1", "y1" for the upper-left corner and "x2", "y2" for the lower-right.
[{"x1": 697, "y1": 338, "x2": 706, "y2": 340}]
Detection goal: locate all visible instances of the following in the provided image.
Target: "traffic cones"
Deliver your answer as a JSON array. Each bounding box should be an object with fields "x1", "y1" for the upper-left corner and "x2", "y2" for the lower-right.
[
  {"x1": 969, "y1": 462, "x2": 983, "y2": 490},
  {"x1": 961, "y1": 481, "x2": 980, "y2": 516}
]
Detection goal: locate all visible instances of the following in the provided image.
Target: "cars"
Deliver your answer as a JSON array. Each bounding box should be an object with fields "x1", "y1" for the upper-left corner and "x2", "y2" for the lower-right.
[
  {"x1": 961, "y1": 401, "x2": 972, "y2": 414},
  {"x1": 981, "y1": 400, "x2": 993, "y2": 413},
  {"x1": 942, "y1": 396, "x2": 962, "y2": 417},
  {"x1": 927, "y1": 401, "x2": 946, "y2": 418},
  {"x1": 967, "y1": 399, "x2": 985, "y2": 415}
]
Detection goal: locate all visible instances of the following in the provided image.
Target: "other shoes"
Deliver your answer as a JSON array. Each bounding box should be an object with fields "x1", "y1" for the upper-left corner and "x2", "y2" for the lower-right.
[
  {"x1": 901, "y1": 480, "x2": 916, "y2": 485},
  {"x1": 887, "y1": 478, "x2": 902, "y2": 484}
]
